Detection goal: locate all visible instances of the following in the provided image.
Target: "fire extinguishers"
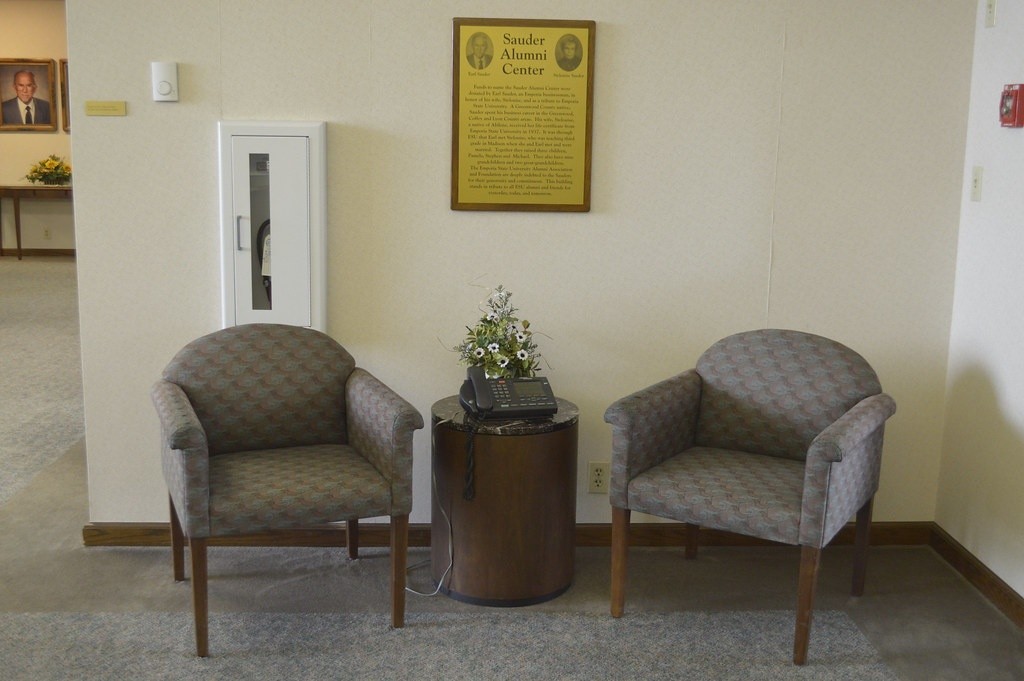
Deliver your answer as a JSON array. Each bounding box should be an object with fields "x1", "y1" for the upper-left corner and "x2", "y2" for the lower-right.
[{"x1": 257, "y1": 219, "x2": 272, "y2": 310}]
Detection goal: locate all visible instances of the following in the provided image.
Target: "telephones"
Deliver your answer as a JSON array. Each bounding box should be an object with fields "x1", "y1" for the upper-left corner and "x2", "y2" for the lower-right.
[{"x1": 458, "y1": 365, "x2": 558, "y2": 423}]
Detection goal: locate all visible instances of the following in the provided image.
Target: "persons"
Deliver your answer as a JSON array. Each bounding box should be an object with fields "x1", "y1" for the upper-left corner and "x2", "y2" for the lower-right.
[
  {"x1": 2, "y1": 69, "x2": 52, "y2": 124},
  {"x1": 559, "y1": 38, "x2": 581, "y2": 71},
  {"x1": 466, "y1": 35, "x2": 493, "y2": 70}
]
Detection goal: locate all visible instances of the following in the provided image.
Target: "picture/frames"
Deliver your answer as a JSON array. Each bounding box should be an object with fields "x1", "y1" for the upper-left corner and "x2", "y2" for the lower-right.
[
  {"x1": 59, "y1": 59, "x2": 70, "y2": 131},
  {"x1": 0, "y1": 58, "x2": 58, "y2": 131}
]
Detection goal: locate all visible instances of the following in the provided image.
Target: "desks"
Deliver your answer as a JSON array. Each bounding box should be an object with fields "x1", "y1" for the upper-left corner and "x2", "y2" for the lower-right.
[
  {"x1": 0, "y1": 185, "x2": 73, "y2": 259},
  {"x1": 430, "y1": 394, "x2": 580, "y2": 605}
]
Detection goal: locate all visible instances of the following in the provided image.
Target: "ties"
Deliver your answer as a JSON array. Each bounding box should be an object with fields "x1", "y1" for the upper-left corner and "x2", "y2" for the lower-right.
[
  {"x1": 479, "y1": 57, "x2": 483, "y2": 69},
  {"x1": 25, "y1": 106, "x2": 32, "y2": 124}
]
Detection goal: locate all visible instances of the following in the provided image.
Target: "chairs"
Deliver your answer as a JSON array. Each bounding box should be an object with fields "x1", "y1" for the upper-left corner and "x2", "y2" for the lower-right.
[
  {"x1": 603, "y1": 329, "x2": 897, "y2": 665},
  {"x1": 150, "y1": 323, "x2": 424, "y2": 658}
]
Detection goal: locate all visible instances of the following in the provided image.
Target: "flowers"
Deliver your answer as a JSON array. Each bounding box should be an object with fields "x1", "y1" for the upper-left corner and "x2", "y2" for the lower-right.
[
  {"x1": 25, "y1": 153, "x2": 72, "y2": 182},
  {"x1": 454, "y1": 286, "x2": 556, "y2": 380}
]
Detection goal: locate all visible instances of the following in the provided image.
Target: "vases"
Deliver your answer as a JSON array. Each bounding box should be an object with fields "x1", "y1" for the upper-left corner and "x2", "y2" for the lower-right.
[{"x1": 43, "y1": 177, "x2": 66, "y2": 184}]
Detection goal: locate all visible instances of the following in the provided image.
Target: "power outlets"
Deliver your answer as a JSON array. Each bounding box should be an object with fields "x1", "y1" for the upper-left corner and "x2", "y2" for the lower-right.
[{"x1": 588, "y1": 461, "x2": 610, "y2": 494}]
